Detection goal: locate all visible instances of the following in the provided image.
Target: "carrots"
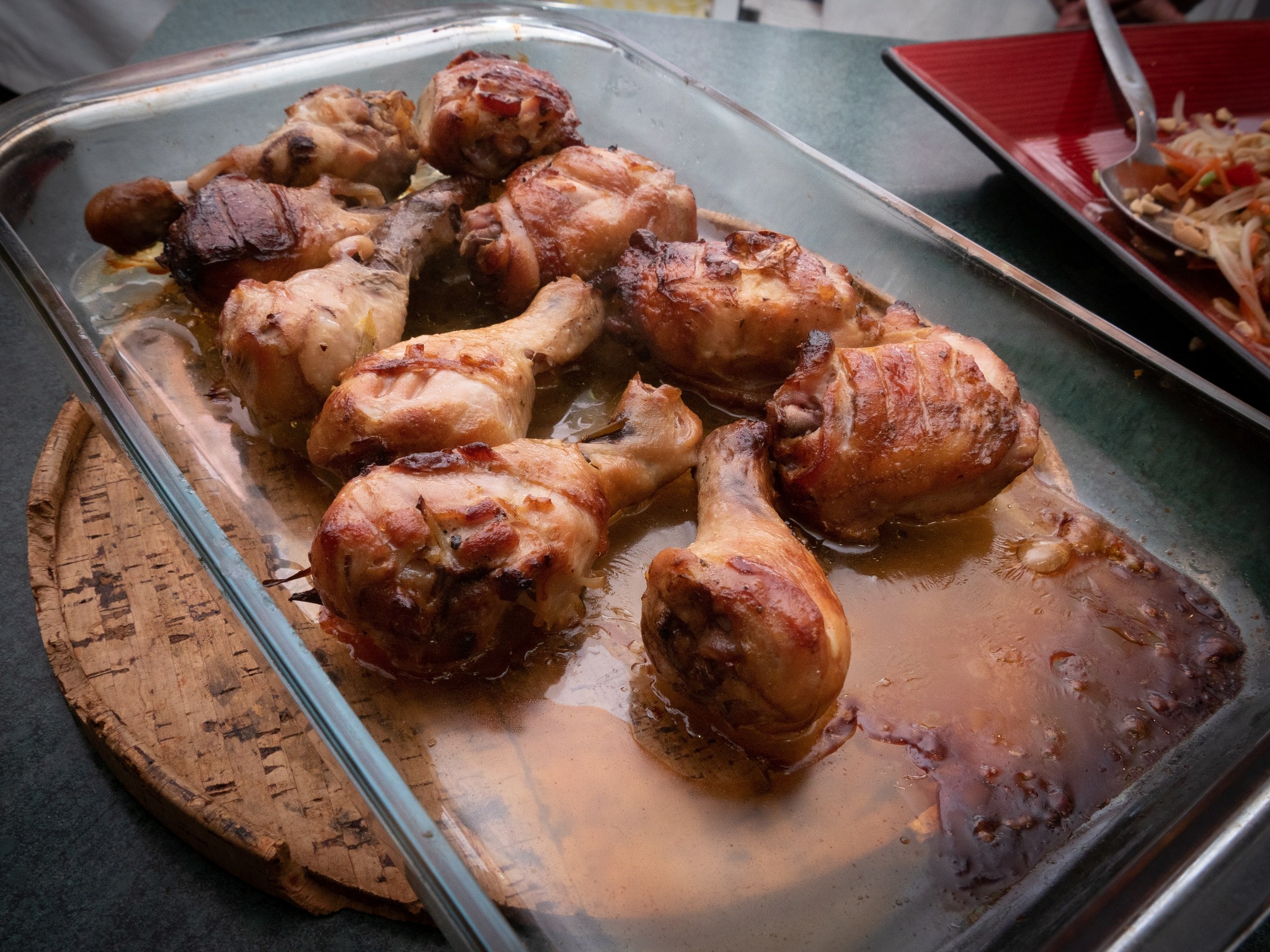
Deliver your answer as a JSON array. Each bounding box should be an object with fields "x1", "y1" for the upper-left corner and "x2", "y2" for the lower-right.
[{"x1": 1150, "y1": 141, "x2": 1270, "y2": 260}]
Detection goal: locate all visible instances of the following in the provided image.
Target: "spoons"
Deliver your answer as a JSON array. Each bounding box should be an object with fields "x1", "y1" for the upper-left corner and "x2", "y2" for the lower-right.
[{"x1": 1086, "y1": 0, "x2": 1219, "y2": 262}]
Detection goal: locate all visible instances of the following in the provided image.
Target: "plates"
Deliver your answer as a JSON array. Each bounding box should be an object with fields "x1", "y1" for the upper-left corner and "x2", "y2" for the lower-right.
[
  {"x1": 0, "y1": 2, "x2": 1268, "y2": 950},
  {"x1": 882, "y1": 18, "x2": 1269, "y2": 385}
]
